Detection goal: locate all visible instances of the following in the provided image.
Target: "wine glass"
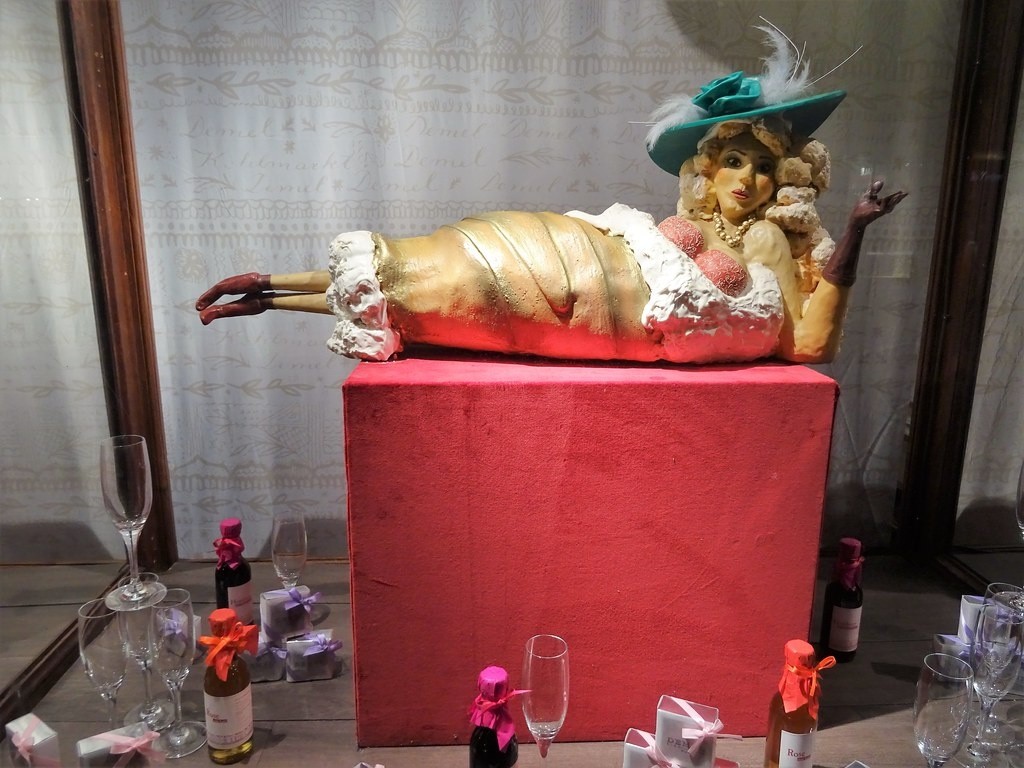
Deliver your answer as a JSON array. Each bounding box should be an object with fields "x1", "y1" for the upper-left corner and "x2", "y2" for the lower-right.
[
  {"x1": 116, "y1": 572, "x2": 176, "y2": 732},
  {"x1": 270, "y1": 510, "x2": 309, "y2": 588},
  {"x1": 912, "y1": 653, "x2": 975, "y2": 768},
  {"x1": 521, "y1": 634, "x2": 571, "y2": 768},
  {"x1": 149, "y1": 588, "x2": 208, "y2": 759},
  {"x1": 78, "y1": 598, "x2": 130, "y2": 732},
  {"x1": 99, "y1": 434, "x2": 168, "y2": 612},
  {"x1": 969, "y1": 583, "x2": 1024, "y2": 745},
  {"x1": 953, "y1": 604, "x2": 1024, "y2": 768}
]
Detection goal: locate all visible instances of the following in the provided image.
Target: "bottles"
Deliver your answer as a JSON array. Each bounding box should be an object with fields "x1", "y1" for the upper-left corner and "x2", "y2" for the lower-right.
[
  {"x1": 203, "y1": 609, "x2": 254, "y2": 765},
  {"x1": 469, "y1": 666, "x2": 519, "y2": 768},
  {"x1": 820, "y1": 537, "x2": 865, "y2": 663},
  {"x1": 214, "y1": 518, "x2": 255, "y2": 627},
  {"x1": 763, "y1": 639, "x2": 820, "y2": 768}
]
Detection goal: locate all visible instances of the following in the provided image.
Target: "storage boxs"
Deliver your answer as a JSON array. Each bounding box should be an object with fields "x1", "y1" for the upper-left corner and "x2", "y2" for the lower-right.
[
  {"x1": 622, "y1": 695, "x2": 738, "y2": 768},
  {"x1": 286, "y1": 629, "x2": 343, "y2": 683},
  {"x1": 238, "y1": 631, "x2": 287, "y2": 682},
  {"x1": 5, "y1": 713, "x2": 60, "y2": 768},
  {"x1": 77, "y1": 722, "x2": 166, "y2": 768},
  {"x1": 260, "y1": 585, "x2": 321, "y2": 640},
  {"x1": 933, "y1": 595, "x2": 1021, "y2": 684}
]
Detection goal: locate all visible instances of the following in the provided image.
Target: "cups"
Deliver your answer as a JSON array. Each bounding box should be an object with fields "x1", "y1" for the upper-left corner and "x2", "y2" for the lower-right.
[{"x1": 1015, "y1": 457, "x2": 1024, "y2": 539}]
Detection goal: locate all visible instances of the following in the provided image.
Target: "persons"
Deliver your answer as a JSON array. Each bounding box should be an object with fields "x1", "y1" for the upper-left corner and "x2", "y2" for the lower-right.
[{"x1": 195, "y1": 111, "x2": 911, "y2": 365}]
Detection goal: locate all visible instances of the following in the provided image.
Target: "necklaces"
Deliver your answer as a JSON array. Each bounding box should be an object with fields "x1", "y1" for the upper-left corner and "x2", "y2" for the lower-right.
[{"x1": 712, "y1": 204, "x2": 757, "y2": 246}]
